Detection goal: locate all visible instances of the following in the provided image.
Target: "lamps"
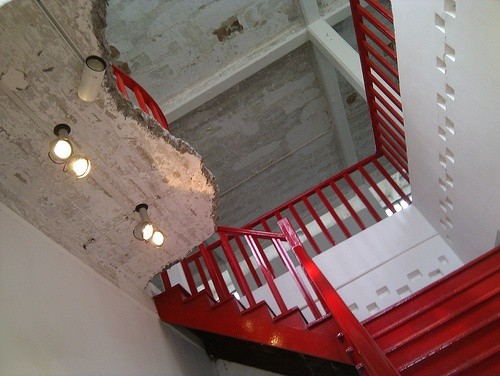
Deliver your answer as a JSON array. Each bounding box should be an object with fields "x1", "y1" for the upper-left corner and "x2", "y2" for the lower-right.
[
  {"x1": 133, "y1": 203, "x2": 164, "y2": 249},
  {"x1": 48, "y1": 123, "x2": 92, "y2": 179},
  {"x1": 78, "y1": 55, "x2": 106, "y2": 103}
]
